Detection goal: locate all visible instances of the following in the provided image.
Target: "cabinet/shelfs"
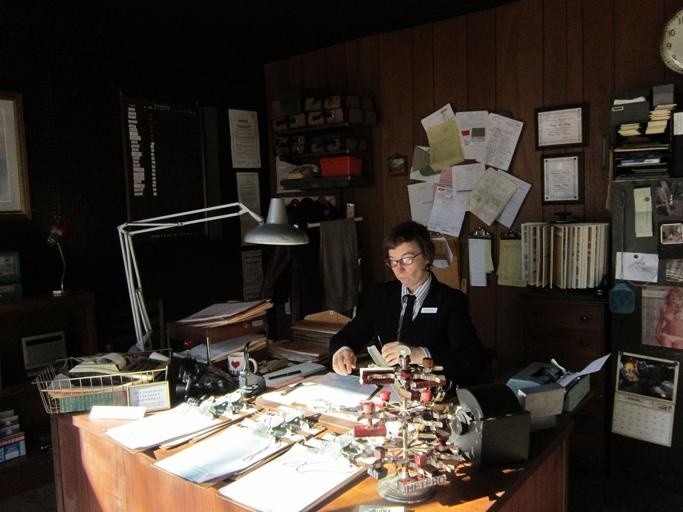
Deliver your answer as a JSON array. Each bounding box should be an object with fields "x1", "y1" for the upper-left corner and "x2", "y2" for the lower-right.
[
  {"x1": 464, "y1": 289, "x2": 618, "y2": 460},
  {"x1": 276, "y1": 122, "x2": 373, "y2": 189},
  {"x1": 0, "y1": 292, "x2": 95, "y2": 500}
]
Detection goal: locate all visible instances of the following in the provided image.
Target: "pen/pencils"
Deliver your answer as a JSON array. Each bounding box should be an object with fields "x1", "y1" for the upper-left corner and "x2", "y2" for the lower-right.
[
  {"x1": 269, "y1": 370, "x2": 301, "y2": 380},
  {"x1": 281, "y1": 382, "x2": 302, "y2": 396},
  {"x1": 366, "y1": 384, "x2": 383, "y2": 401}
]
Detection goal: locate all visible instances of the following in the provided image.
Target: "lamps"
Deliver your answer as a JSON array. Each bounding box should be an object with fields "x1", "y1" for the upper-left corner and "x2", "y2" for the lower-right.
[
  {"x1": 47, "y1": 216, "x2": 76, "y2": 297},
  {"x1": 116, "y1": 198, "x2": 311, "y2": 352}
]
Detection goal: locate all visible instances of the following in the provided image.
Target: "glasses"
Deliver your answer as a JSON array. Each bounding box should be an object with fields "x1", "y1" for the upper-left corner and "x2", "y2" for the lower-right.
[{"x1": 384, "y1": 250, "x2": 425, "y2": 267}]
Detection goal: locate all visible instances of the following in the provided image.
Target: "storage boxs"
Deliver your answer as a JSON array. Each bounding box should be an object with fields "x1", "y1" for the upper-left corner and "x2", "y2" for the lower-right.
[
  {"x1": 610, "y1": 349, "x2": 681, "y2": 449},
  {"x1": 319, "y1": 155, "x2": 363, "y2": 177}
]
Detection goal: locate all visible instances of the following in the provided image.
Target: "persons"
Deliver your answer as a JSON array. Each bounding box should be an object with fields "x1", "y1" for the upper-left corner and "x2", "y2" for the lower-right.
[
  {"x1": 656, "y1": 288, "x2": 683, "y2": 350},
  {"x1": 329, "y1": 222, "x2": 482, "y2": 399}
]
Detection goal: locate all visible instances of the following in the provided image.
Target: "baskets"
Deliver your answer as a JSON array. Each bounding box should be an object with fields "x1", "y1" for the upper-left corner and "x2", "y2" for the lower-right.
[{"x1": 32, "y1": 348, "x2": 174, "y2": 415}]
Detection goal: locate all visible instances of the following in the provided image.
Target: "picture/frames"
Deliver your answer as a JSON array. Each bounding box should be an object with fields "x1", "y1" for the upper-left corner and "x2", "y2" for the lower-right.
[
  {"x1": 0, "y1": 94, "x2": 31, "y2": 220},
  {"x1": 535, "y1": 101, "x2": 589, "y2": 149},
  {"x1": 540, "y1": 153, "x2": 584, "y2": 206}
]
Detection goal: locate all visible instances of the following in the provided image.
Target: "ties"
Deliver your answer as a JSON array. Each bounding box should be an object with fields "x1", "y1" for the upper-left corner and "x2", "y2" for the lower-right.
[{"x1": 399, "y1": 295, "x2": 416, "y2": 340}]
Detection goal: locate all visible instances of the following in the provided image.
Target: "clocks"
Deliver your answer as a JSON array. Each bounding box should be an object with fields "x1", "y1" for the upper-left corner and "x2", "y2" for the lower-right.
[{"x1": 656, "y1": 7, "x2": 683, "y2": 75}]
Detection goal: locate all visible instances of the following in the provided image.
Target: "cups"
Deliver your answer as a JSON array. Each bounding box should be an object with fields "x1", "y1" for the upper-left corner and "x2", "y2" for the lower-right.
[{"x1": 227, "y1": 352, "x2": 258, "y2": 377}]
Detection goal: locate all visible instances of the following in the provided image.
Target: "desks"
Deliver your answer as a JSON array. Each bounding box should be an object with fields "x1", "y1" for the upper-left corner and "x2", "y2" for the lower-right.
[{"x1": 50, "y1": 378, "x2": 569, "y2": 512}]
